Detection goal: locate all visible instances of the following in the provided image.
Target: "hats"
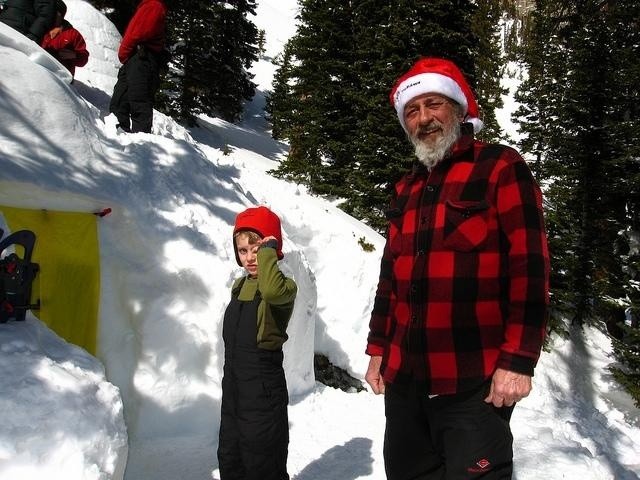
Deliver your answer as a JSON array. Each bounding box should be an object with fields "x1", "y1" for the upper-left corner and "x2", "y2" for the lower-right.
[
  {"x1": 391, "y1": 56, "x2": 485, "y2": 137},
  {"x1": 230, "y1": 205, "x2": 286, "y2": 269}
]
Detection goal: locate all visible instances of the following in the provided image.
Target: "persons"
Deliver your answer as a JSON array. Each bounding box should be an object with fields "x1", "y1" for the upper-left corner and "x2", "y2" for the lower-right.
[
  {"x1": 365, "y1": 58, "x2": 550, "y2": 480},
  {"x1": 217, "y1": 206, "x2": 297, "y2": 480},
  {"x1": 0, "y1": 0, "x2": 89, "y2": 85},
  {"x1": 110, "y1": 0, "x2": 172, "y2": 134}
]
